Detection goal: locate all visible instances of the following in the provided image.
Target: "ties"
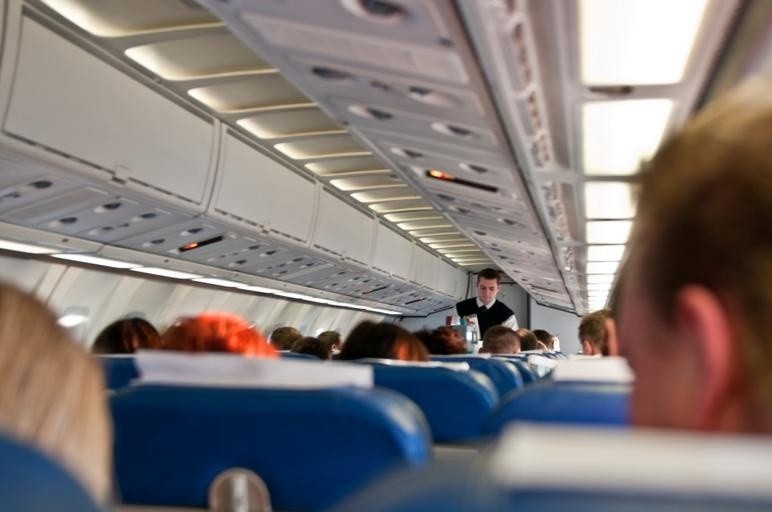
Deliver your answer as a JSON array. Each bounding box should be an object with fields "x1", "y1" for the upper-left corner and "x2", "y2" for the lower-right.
[{"x1": 479, "y1": 305, "x2": 488, "y2": 310}]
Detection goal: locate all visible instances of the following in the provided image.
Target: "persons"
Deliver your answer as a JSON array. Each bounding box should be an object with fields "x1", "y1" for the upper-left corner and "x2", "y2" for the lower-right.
[
  {"x1": 0, "y1": 281, "x2": 114, "y2": 512},
  {"x1": 579, "y1": 71, "x2": 772, "y2": 432},
  {"x1": 452, "y1": 267, "x2": 518, "y2": 351},
  {"x1": 93, "y1": 314, "x2": 554, "y2": 362}
]
